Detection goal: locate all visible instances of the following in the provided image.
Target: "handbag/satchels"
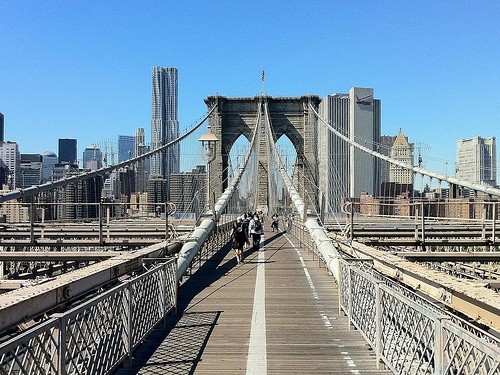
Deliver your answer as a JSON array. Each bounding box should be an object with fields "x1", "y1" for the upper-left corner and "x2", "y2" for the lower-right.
[{"x1": 231, "y1": 231, "x2": 239, "y2": 248}]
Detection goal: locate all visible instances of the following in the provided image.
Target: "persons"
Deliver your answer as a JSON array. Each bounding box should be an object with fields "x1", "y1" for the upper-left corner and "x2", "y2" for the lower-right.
[
  {"x1": 231, "y1": 223, "x2": 250, "y2": 264},
  {"x1": 285, "y1": 214, "x2": 294, "y2": 234},
  {"x1": 272, "y1": 212, "x2": 279, "y2": 233},
  {"x1": 236, "y1": 210, "x2": 265, "y2": 249}
]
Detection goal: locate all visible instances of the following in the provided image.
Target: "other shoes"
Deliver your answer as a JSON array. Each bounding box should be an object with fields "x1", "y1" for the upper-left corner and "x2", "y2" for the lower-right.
[
  {"x1": 244, "y1": 246, "x2": 248, "y2": 249},
  {"x1": 240, "y1": 257, "x2": 245, "y2": 262},
  {"x1": 237, "y1": 261, "x2": 240, "y2": 266}
]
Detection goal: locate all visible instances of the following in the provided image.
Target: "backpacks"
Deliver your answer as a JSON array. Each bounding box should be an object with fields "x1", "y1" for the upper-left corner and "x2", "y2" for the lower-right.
[{"x1": 253, "y1": 219, "x2": 262, "y2": 231}]
